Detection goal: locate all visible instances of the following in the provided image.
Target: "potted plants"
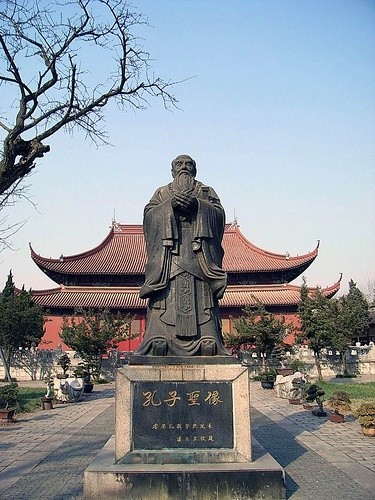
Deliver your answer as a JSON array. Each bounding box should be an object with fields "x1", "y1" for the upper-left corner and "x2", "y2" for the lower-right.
[
  {"x1": 305, "y1": 384, "x2": 327, "y2": 416},
  {"x1": 259, "y1": 366, "x2": 277, "y2": 390},
  {"x1": 328, "y1": 392, "x2": 351, "y2": 423},
  {"x1": 41, "y1": 371, "x2": 56, "y2": 410},
  {"x1": 73, "y1": 360, "x2": 99, "y2": 393},
  {"x1": 0, "y1": 383, "x2": 19, "y2": 419},
  {"x1": 289, "y1": 377, "x2": 305, "y2": 405},
  {"x1": 355, "y1": 402, "x2": 375, "y2": 437},
  {"x1": 56, "y1": 352, "x2": 72, "y2": 378}
]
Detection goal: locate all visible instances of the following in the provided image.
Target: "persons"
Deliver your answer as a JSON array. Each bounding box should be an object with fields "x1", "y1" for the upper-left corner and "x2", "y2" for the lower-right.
[{"x1": 131, "y1": 155, "x2": 233, "y2": 357}]
